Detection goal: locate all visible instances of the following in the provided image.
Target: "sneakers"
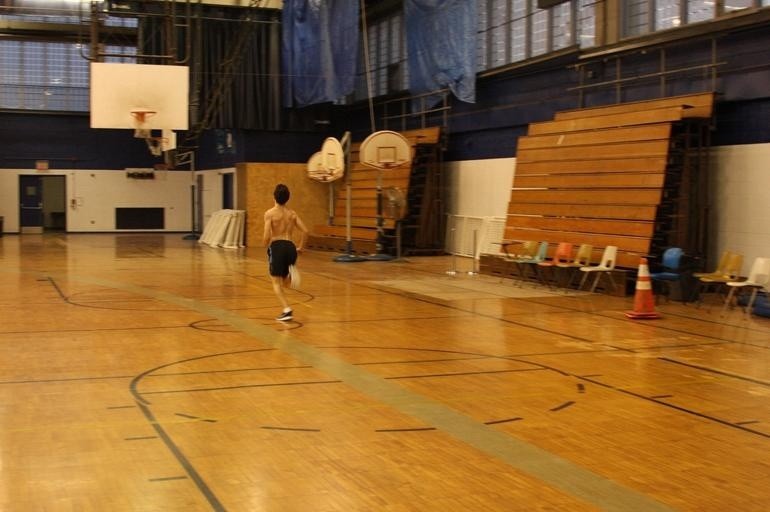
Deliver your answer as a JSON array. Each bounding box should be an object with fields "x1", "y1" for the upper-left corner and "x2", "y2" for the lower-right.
[
  {"x1": 275, "y1": 311, "x2": 292, "y2": 321},
  {"x1": 288, "y1": 265, "x2": 300, "y2": 287}
]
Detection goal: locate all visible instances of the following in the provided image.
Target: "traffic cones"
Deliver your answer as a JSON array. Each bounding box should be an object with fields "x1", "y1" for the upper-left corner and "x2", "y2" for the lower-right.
[{"x1": 625, "y1": 258, "x2": 663, "y2": 319}]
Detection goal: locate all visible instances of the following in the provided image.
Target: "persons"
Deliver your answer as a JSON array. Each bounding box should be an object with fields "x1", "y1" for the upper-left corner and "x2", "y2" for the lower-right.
[{"x1": 261, "y1": 184, "x2": 309, "y2": 322}]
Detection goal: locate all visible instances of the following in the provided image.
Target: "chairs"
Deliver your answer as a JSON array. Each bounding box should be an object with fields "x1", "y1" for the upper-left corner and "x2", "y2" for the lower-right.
[{"x1": 492, "y1": 238, "x2": 769, "y2": 324}]
